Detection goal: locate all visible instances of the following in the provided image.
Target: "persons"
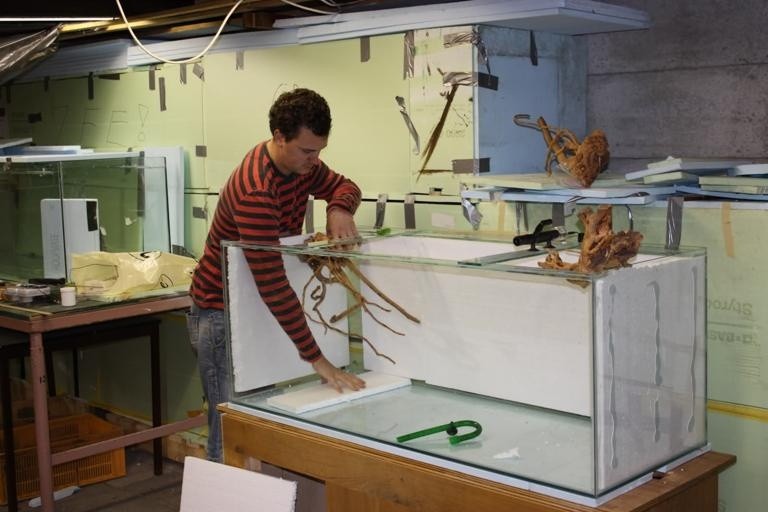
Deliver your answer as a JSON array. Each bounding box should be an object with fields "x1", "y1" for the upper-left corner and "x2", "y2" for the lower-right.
[{"x1": 183, "y1": 87, "x2": 368, "y2": 465}]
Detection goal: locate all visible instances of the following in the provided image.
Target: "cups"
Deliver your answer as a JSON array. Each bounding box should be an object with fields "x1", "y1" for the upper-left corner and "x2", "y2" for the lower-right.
[{"x1": 60, "y1": 286, "x2": 76, "y2": 306}]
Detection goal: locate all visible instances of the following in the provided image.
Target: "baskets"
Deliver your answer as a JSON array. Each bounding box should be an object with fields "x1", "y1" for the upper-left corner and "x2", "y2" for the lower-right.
[{"x1": 2, "y1": 397, "x2": 126, "y2": 506}]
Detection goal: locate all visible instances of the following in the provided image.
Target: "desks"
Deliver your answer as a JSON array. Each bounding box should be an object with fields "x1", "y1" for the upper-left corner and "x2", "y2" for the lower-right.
[
  {"x1": 214, "y1": 401, "x2": 738, "y2": 512},
  {"x1": 0, "y1": 293, "x2": 208, "y2": 512}
]
